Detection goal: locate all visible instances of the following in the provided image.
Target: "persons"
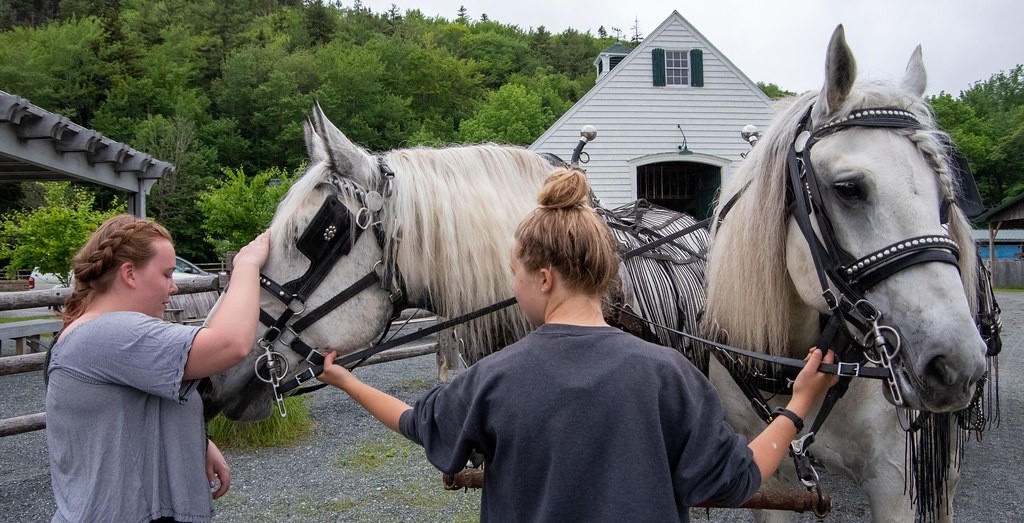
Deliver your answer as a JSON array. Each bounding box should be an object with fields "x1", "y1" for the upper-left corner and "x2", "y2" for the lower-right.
[
  {"x1": 44, "y1": 213, "x2": 270, "y2": 523},
  {"x1": 316, "y1": 169, "x2": 839, "y2": 523}
]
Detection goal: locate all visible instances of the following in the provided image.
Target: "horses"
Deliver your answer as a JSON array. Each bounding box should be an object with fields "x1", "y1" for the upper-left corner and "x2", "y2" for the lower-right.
[
  {"x1": 197, "y1": 96, "x2": 711, "y2": 422},
  {"x1": 696, "y1": 23, "x2": 1004, "y2": 522}
]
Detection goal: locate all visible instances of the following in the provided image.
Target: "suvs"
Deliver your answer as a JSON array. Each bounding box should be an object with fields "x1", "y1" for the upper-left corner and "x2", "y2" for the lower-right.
[{"x1": 29, "y1": 256, "x2": 220, "y2": 315}]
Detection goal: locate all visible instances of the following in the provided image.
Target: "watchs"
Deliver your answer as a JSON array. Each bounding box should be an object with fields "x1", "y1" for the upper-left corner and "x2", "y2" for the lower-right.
[{"x1": 770, "y1": 406, "x2": 804, "y2": 434}]
